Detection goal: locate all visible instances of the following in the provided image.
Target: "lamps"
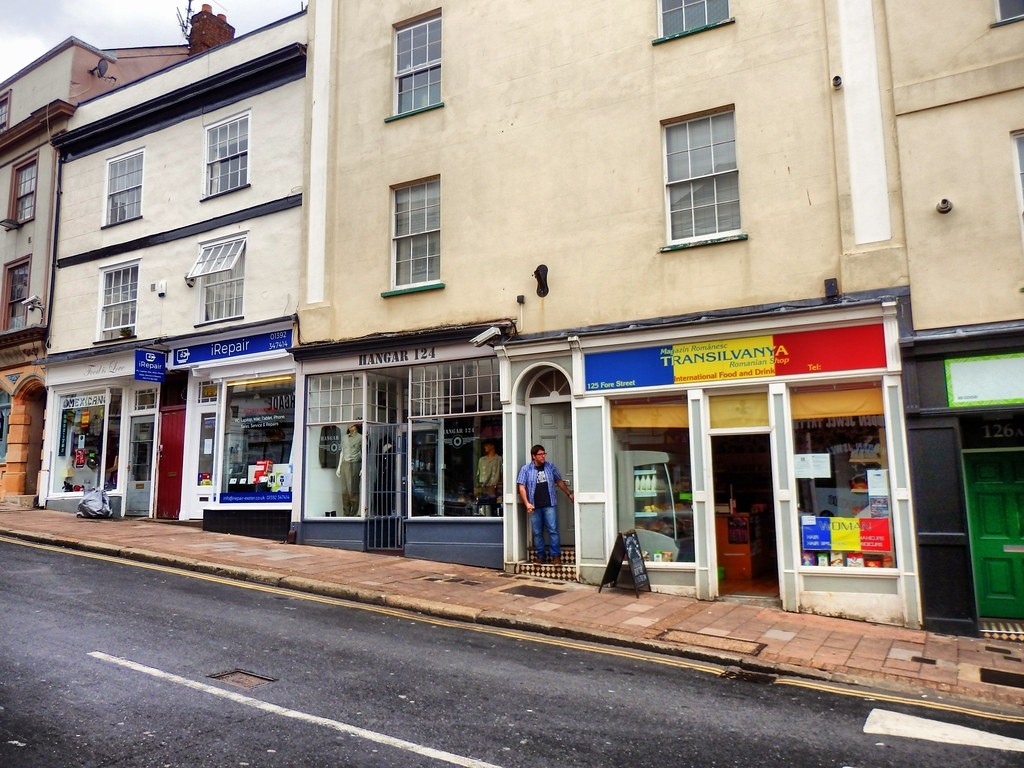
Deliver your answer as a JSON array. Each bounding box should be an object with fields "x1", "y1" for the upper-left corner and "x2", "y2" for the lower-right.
[{"x1": 0, "y1": 218, "x2": 24, "y2": 230}]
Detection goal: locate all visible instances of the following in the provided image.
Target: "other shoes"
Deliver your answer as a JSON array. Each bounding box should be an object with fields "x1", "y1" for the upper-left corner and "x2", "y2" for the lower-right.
[
  {"x1": 533, "y1": 559, "x2": 546, "y2": 565},
  {"x1": 554, "y1": 556, "x2": 561, "y2": 565}
]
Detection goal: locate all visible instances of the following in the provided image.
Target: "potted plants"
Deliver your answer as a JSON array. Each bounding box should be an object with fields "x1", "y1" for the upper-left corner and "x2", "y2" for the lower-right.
[{"x1": 119, "y1": 324, "x2": 133, "y2": 337}]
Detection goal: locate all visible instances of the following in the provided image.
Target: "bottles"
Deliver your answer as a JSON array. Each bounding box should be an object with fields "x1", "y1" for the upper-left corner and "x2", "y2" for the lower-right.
[
  {"x1": 634, "y1": 475, "x2": 640, "y2": 492},
  {"x1": 640, "y1": 476, "x2": 646, "y2": 492},
  {"x1": 646, "y1": 475, "x2": 651, "y2": 493},
  {"x1": 651, "y1": 475, "x2": 666, "y2": 492}
]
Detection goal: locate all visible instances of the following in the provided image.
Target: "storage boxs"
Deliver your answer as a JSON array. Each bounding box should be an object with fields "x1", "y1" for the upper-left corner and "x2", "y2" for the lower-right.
[
  {"x1": 801, "y1": 550, "x2": 817, "y2": 566},
  {"x1": 267, "y1": 471, "x2": 286, "y2": 487},
  {"x1": 817, "y1": 553, "x2": 829, "y2": 566},
  {"x1": 254, "y1": 460, "x2": 274, "y2": 478},
  {"x1": 830, "y1": 551, "x2": 846, "y2": 566},
  {"x1": 845, "y1": 552, "x2": 865, "y2": 567},
  {"x1": 864, "y1": 560, "x2": 882, "y2": 568}
]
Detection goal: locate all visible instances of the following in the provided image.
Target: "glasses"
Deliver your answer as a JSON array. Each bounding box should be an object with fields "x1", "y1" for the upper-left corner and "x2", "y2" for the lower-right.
[{"x1": 536, "y1": 453, "x2": 547, "y2": 456}]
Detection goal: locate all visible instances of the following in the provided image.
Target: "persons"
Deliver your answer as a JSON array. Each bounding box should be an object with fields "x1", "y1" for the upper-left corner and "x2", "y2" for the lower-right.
[
  {"x1": 335, "y1": 424, "x2": 364, "y2": 517},
  {"x1": 516, "y1": 445, "x2": 575, "y2": 566},
  {"x1": 475, "y1": 436, "x2": 503, "y2": 498}
]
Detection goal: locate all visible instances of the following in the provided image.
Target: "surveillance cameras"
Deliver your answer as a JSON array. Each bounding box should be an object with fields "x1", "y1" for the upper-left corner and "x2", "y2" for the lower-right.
[
  {"x1": 187, "y1": 277, "x2": 195, "y2": 287},
  {"x1": 21, "y1": 296, "x2": 43, "y2": 309},
  {"x1": 158, "y1": 283, "x2": 166, "y2": 297},
  {"x1": 833, "y1": 76, "x2": 842, "y2": 90},
  {"x1": 936, "y1": 199, "x2": 952, "y2": 213},
  {"x1": 29, "y1": 303, "x2": 35, "y2": 310},
  {"x1": 468, "y1": 328, "x2": 502, "y2": 347}
]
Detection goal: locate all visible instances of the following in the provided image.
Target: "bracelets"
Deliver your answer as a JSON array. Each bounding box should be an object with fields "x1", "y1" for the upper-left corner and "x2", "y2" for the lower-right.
[{"x1": 568, "y1": 492, "x2": 572, "y2": 496}]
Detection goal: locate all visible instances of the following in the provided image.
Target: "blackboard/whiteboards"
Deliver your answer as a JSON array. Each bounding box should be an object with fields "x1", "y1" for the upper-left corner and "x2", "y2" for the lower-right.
[{"x1": 601, "y1": 528, "x2": 649, "y2": 589}]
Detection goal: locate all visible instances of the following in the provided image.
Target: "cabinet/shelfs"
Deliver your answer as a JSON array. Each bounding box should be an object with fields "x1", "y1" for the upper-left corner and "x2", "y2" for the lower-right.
[
  {"x1": 728, "y1": 469, "x2": 772, "y2": 494},
  {"x1": 712, "y1": 468, "x2": 728, "y2": 494},
  {"x1": 616, "y1": 450, "x2": 692, "y2": 545},
  {"x1": 849, "y1": 427, "x2": 889, "y2": 493}
]
